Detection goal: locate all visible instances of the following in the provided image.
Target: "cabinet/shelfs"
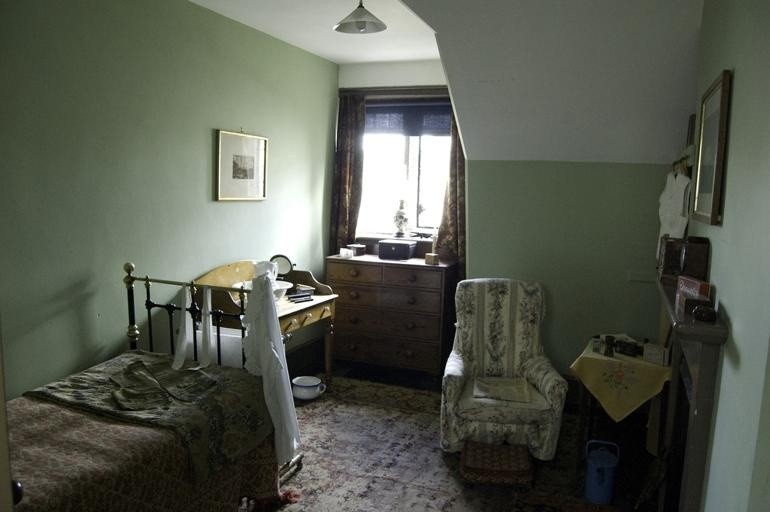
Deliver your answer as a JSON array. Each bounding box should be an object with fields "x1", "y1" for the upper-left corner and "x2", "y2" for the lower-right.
[{"x1": 325, "y1": 254, "x2": 458, "y2": 394}]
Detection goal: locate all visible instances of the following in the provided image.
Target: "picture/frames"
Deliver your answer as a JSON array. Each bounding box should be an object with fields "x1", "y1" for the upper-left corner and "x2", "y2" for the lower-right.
[
  {"x1": 216, "y1": 129, "x2": 269, "y2": 202},
  {"x1": 690, "y1": 69, "x2": 735, "y2": 228}
]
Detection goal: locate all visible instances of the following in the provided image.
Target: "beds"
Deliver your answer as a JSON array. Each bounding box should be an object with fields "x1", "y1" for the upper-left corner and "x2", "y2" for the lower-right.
[{"x1": 0, "y1": 261, "x2": 306, "y2": 512}]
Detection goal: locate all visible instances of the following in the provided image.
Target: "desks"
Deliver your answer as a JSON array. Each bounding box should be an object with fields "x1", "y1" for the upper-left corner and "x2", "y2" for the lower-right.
[
  {"x1": 191, "y1": 260, "x2": 339, "y2": 381},
  {"x1": 571, "y1": 334, "x2": 664, "y2": 457}
]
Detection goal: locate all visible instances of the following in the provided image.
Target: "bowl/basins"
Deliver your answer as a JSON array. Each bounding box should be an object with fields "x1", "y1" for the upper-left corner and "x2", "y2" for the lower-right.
[
  {"x1": 291, "y1": 375, "x2": 327, "y2": 400},
  {"x1": 232, "y1": 280, "x2": 293, "y2": 302}
]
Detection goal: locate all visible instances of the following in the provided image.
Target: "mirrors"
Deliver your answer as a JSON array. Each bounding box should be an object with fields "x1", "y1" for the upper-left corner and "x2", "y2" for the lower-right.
[{"x1": 270, "y1": 253, "x2": 295, "y2": 277}]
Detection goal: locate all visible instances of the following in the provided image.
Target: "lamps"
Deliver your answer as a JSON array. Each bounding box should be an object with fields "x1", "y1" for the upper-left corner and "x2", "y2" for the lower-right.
[{"x1": 332, "y1": 0, "x2": 386, "y2": 35}]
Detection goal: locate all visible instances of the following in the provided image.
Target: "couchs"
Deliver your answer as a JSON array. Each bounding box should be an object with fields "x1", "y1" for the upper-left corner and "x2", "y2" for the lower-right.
[{"x1": 439, "y1": 278, "x2": 570, "y2": 460}]
